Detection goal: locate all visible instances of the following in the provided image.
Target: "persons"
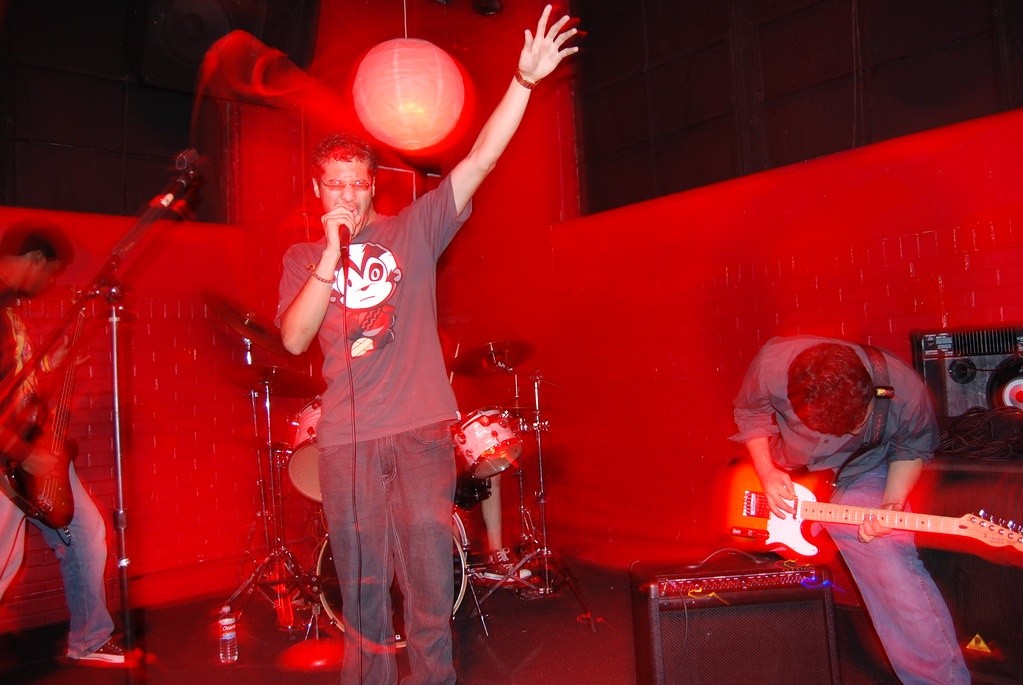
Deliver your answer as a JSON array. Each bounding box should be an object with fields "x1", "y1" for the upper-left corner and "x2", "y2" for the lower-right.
[
  {"x1": 0, "y1": 225, "x2": 159, "y2": 670},
  {"x1": 441, "y1": 327, "x2": 533, "y2": 582},
  {"x1": 278, "y1": 3, "x2": 580, "y2": 685},
  {"x1": 726, "y1": 335, "x2": 974, "y2": 685}
]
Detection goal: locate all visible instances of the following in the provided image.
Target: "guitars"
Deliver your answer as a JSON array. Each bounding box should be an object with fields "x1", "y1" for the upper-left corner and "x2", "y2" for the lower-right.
[
  {"x1": 706, "y1": 462, "x2": 1023, "y2": 559},
  {"x1": 0, "y1": 357, "x2": 75, "y2": 530}
]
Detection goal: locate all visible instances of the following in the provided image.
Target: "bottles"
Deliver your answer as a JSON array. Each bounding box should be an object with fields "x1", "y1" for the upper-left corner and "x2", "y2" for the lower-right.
[{"x1": 219, "y1": 606, "x2": 238, "y2": 663}]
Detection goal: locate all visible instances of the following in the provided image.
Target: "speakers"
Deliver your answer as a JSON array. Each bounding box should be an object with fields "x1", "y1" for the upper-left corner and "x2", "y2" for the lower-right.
[
  {"x1": 630, "y1": 567, "x2": 843, "y2": 685},
  {"x1": 910, "y1": 324, "x2": 1023, "y2": 426}
]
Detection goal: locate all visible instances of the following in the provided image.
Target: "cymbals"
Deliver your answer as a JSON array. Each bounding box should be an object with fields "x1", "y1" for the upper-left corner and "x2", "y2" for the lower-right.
[
  {"x1": 450, "y1": 338, "x2": 536, "y2": 378},
  {"x1": 199, "y1": 289, "x2": 292, "y2": 356}
]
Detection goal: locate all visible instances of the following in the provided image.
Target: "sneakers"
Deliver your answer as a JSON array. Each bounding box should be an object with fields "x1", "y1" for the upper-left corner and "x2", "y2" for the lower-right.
[
  {"x1": 483, "y1": 550, "x2": 531, "y2": 582},
  {"x1": 66, "y1": 643, "x2": 126, "y2": 664}
]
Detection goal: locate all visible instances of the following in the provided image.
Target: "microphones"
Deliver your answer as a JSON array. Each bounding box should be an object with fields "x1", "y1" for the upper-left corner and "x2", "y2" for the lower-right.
[{"x1": 339, "y1": 223, "x2": 350, "y2": 280}]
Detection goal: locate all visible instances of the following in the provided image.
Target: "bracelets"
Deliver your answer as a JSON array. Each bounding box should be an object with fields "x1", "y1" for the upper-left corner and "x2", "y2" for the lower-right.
[
  {"x1": 515, "y1": 70, "x2": 542, "y2": 89},
  {"x1": 308, "y1": 264, "x2": 337, "y2": 284}
]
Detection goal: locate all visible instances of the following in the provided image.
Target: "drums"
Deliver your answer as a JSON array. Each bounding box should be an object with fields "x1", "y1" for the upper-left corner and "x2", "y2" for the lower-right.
[
  {"x1": 315, "y1": 508, "x2": 471, "y2": 647},
  {"x1": 286, "y1": 397, "x2": 325, "y2": 505},
  {"x1": 452, "y1": 404, "x2": 523, "y2": 478}
]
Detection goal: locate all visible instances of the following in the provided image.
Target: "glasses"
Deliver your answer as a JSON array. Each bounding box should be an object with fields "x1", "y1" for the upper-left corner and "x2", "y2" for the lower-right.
[{"x1": 316, "y1": 176, "x2": 374, "y2": 192}]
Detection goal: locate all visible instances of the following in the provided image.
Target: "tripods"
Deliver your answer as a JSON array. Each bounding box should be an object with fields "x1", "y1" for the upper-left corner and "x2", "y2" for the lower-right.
[
  {"x1": 224, "y1": 337, "x2": 317, "y2": 623},
  {"x1": 470, "y1": 346, "x2": 597, "y2": 635}
]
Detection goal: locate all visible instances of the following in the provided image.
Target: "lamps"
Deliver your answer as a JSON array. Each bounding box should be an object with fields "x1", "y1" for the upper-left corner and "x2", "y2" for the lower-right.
[{"x1": 351, "y1": 0, "x2": 465, "y2": 149}]
